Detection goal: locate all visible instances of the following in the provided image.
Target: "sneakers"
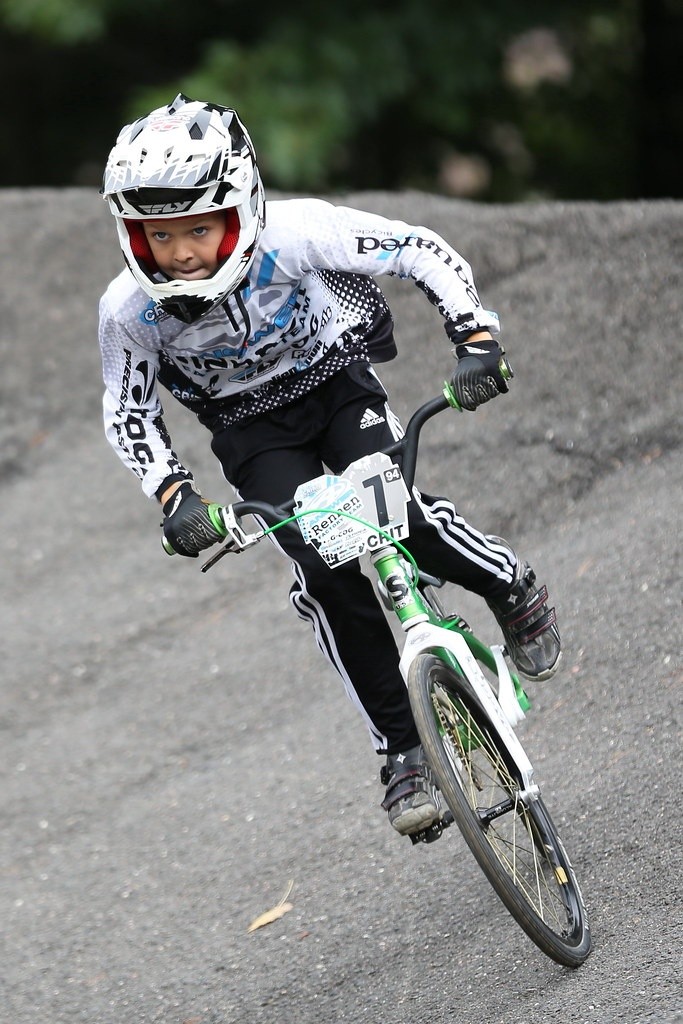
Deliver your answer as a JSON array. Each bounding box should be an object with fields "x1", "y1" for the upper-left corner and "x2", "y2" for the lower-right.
[
  {"x1": 381, "y1": 741, "x2": 441, "y2": 835},
  {"x1": 483, "y1": 535, "x2": 562, "y2": 682}
]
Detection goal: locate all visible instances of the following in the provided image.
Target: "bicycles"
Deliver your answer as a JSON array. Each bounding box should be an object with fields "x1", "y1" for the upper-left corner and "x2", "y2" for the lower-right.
[{"x1": 154, "y1": 345, "x2": 594, "y2": 969}]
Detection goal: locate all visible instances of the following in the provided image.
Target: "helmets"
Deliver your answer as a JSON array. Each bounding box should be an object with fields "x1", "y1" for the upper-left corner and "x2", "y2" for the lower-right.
[{"x1": 101, "y1": 92, "x2": 266, "y2": 324}]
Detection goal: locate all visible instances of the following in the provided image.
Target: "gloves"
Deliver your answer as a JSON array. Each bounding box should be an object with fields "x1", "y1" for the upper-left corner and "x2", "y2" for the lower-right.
[
  {"x1": 162, "y1": 478, "x2": 234, "y2": 558},
  {"x1": 451, "y1": 340, "x2": 509, "y2": 411}
]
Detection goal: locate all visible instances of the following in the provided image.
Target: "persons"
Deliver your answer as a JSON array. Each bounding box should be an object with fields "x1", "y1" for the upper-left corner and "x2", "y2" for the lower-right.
[{"x1": 98, "y1": 93, "x2": 562, "y2": 834}]
